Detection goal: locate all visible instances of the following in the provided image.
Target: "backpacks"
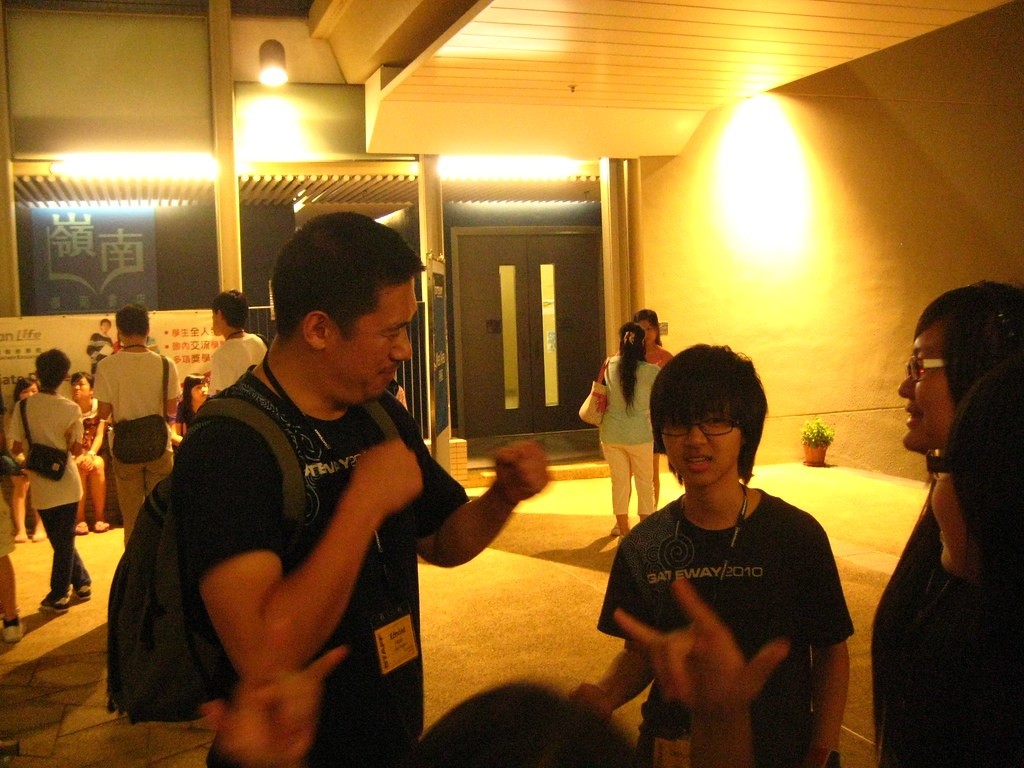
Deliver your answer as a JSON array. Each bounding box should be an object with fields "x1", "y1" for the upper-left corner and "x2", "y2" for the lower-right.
[{"x1": 106, "y1": 398, "x2": 403, "y2": 725}]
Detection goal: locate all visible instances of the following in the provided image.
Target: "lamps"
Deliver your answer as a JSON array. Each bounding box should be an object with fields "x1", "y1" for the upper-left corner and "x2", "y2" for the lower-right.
[{"x1": 258, "y1": 40, "x2": 288, "y2": 88}]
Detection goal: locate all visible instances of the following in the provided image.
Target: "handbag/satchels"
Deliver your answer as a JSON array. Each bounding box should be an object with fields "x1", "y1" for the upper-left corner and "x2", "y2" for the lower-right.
[
  {"x1": 72, "y1": 417, "x2": 106, "y2": 454},
  {"x1": 577, "y1": 359, "x2": 607, "y2": 426},
  {"x1": 112, "y1": 415, "x2": 168, "y2": 465},
  {"x1": 24, "y1": 442, "x2": 68, "y2": 482}
]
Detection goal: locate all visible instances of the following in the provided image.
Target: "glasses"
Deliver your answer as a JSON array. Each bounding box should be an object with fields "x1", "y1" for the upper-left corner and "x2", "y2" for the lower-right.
[
  {"x1": 657, "y1": 413, "x2": 745, "y2": 437},
  {"x1": 905, "y1": 356, "x2": 948, "y2": 382},
  {"x1": 926, "y1": 450, "x2": 959, "y2": 481}
]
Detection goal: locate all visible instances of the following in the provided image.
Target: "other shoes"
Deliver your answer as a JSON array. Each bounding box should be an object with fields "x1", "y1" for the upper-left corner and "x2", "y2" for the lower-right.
[
  {"x1": 13, "y1": 532, "x2": 28, "y2": 544},
  {"x1": 73, "y1": 520, "x2": 89, "y2": 534},
  {"x1": 611, "y1": 522, "x2": 621, "y2": 536},
  {"x1": 95, "y1": 522, "x2": 110, "y2": 532},
  {"x1": 32, "y1": 532, "x2": 48, "y2": 542}
]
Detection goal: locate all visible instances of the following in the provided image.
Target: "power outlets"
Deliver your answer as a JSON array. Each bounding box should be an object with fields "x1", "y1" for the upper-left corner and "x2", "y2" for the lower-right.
[{"x1": 658, "y1": 322, "x2": 668, "y2": 337}]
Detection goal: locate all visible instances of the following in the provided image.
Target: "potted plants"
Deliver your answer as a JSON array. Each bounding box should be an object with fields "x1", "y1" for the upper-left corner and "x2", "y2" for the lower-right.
[{"x1": 803, "y1": 415, "x2": 835, "y2": 466}]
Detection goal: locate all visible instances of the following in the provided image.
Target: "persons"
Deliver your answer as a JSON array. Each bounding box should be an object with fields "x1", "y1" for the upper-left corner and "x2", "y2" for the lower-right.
[
  {"x1": 871, "y1": 282, "x2": 1024, "y2": 768},
  {"x1": 68, "y1": 371, "x2": 111, "y2": 536},
  {"x1": 0, "y1": 393, "x2": 26, "y2": 645},
  {"x1": 176, "y1": 373, "x2": 208, "y2": 437},
  {"x1": 599, "y1": 321, "x2": 658, "y2": 547},
  {"x1": 207, "y1": 290, "x2": 267, "y2": 398},
  {"x1": 588, "y1": 344, "x2": 853, "y2": 768},
  {"x1": 109, "y1": 212, "x2": 550, "y2": 768},
  {"x1": 0, "y1": 363, "x2": 217, "y2": 543},
  {"x1": 199, "y1": 573, "x2": 787, "y2": 768},
  {"x1": 87, "y1": 319, "x2": 114, "y2": 390},
  {"x1": 612, "y1": 310, "x2": 675, "y2": 537},
  {"x1": 384, "y1": 378, "x2": 408, "y2": 407},
  {"x1": 98, "y1": 304, "x2": 177, "y2": 547},
  {"x1": 14, "y1": 348, "x2": 90, "y2": 613}
]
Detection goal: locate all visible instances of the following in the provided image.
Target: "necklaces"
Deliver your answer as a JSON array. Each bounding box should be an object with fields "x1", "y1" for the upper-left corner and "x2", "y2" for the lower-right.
[{"x1": 122, "y1": 344, "x2": 147, "y2": 349}]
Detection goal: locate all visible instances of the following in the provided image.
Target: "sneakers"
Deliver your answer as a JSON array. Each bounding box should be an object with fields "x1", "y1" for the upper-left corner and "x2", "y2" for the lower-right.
[
  {"x1": 2, "y1": 620, "x2": 28, "y2": 643},
  {"x1": 40, "y1": 594, "x2": 70, "y2": 613},
  {"x1": 71, "y1": 585, "x2": 91, "y2": 601}
]
